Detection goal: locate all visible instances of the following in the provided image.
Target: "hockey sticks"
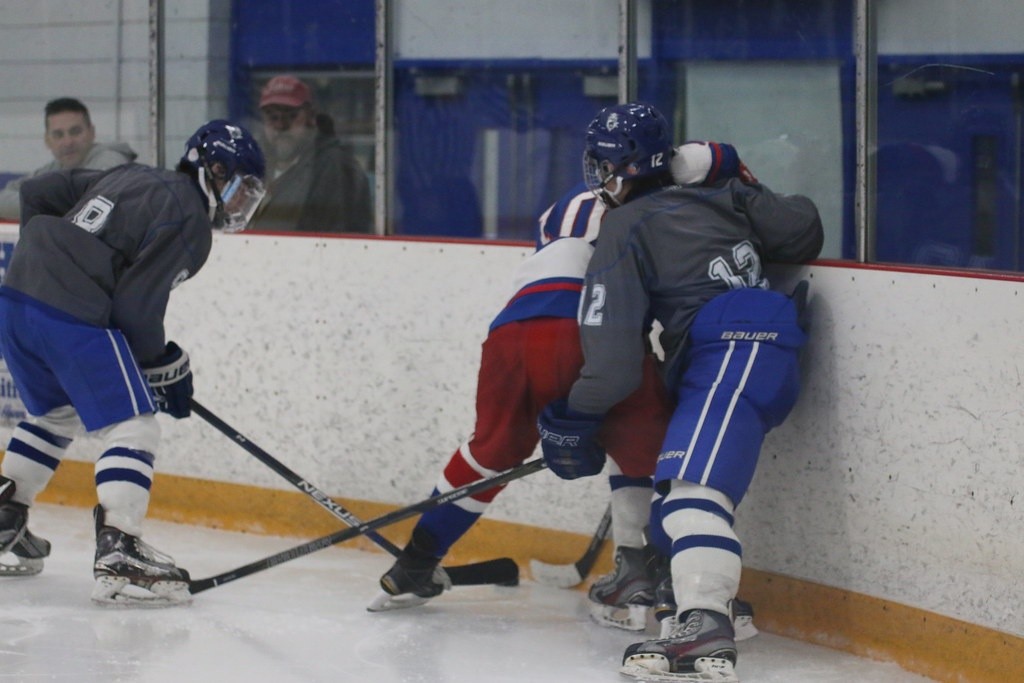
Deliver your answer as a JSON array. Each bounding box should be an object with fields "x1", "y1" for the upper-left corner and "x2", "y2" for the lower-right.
[
  {"x1": 529, "y1": 500, "x2": 612, "y2": 590},
  {"x1": 150, "y1": 456, "x2": 549, "y2": 602},
  {"x1": 189, "y1": 400, "x2": 519, "y2": 588}
]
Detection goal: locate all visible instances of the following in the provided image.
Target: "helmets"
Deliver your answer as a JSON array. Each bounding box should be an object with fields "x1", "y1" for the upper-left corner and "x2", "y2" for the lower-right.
[
  {"x1": 582, "y1": 101, "x2": 674, "y2": 210},
  {"x1": 184, "y1": 119, "x2": 266, "y2": 228}
]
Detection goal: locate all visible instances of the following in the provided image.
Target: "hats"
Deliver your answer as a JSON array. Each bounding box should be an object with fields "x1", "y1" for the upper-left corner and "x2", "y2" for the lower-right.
[{"x1": 259, "y1": 76, "x2": 312, "y2": 109}]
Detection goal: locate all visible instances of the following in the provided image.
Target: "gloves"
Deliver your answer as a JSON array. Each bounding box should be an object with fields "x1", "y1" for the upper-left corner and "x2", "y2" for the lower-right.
[
  {"x1": 136, "y1": 340, "x2": 194, "y2": 419},
  {"x1": 537, "y1": 401, "x2": 607, "y2": 481}
]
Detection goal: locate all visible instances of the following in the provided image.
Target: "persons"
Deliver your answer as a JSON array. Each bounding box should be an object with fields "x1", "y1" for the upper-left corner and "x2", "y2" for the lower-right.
[
  {"x1": 536, "y1": 102, "x2": 825, "y2": 683},
  {"x1": 243, "y1": 76, "x2": 371, "y2": 234},
  {"x1": 366, "y1": 141, "x2": 758, "y2": 642},
  {"x1": 0, "y1": 97, "x2": 138, "y2": 220},
  {"x1": 0, "y1": 120, "x2": 266, "y2": 608}
]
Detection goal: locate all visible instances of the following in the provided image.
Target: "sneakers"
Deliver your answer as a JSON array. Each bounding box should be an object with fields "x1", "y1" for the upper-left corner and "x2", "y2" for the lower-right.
[
  {"x1": 0, "y1": 474, "x2": 52, "y2": 576},
  {"x1": 619, "y1": 600, "x2": 740, "y2": 683},
  {"x1": 587, "y1": 544, "x2": 654, "y2": 633},
  {"x1": 366, "y1": 529, "x2": 444, "y2": 612},
  {"x1": 643, "y1": 543, "x2": 759, "y2": 643},
  {"x1": 90, "y1": 501, "x2": 193, "y2": 608}
]
